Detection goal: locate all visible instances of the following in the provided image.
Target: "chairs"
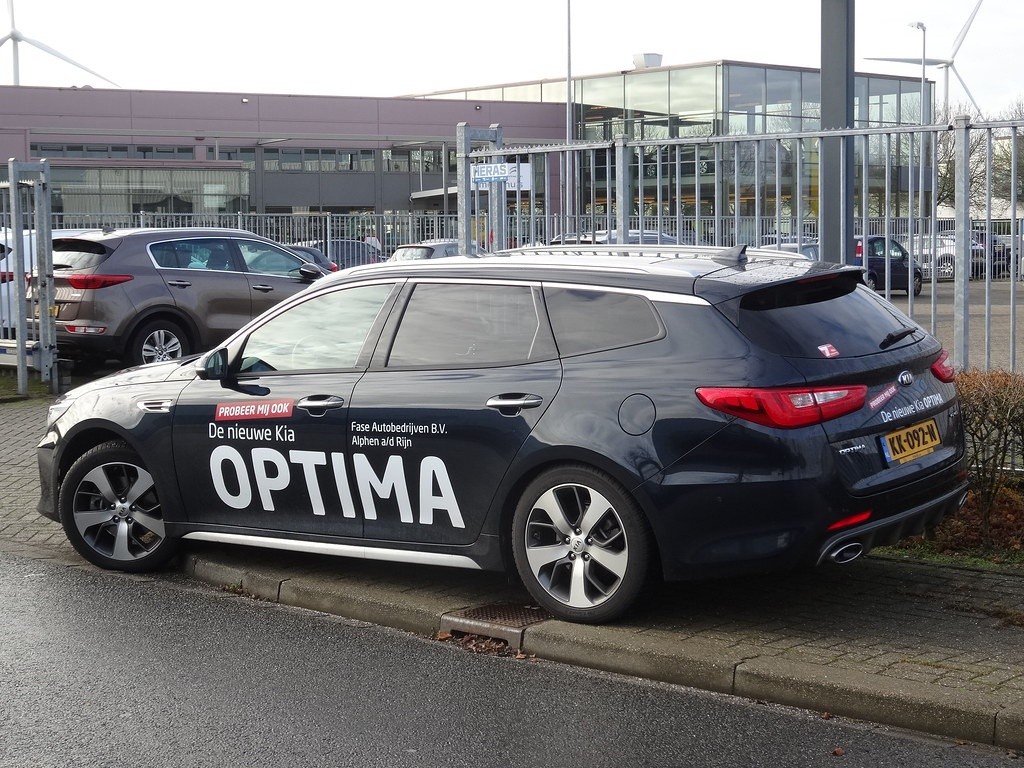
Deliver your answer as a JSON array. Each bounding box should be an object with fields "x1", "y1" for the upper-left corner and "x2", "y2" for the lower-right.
[
  {"x1": 170, "y1": 248, "x2": 193, "y2": 268},
  {"x1": 206, "y1": 248, "x2": 227, "y2": 270}
]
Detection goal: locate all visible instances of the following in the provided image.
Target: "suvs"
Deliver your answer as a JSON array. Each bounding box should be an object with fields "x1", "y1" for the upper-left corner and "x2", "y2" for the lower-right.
[
  {"x1": 35, "y1": 239, "x2": 969, "y2": 626},
  {"x1": 572, "y1": 229, "x2": 687, "y2": 250},
  {"x1": 904, "y1": 234, "x2": 1024, "y2": 282},
  {"x1": 25, "y1": 227, "x2": 334, "y2": 376},
  {"x1": 0, "y1": 224, "x2": 108, "y2": 341}
]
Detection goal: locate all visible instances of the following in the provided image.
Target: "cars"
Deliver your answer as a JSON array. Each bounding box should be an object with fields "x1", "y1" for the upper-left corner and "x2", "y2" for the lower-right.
[
  {"x1": 758, "y1": 244, "x2": 820, "y2": 265},
  {"x1": 287, "y1": 239, "x2": 381, "y2": 271},
  {"x1": 385, "y1": 242, "x2": 489, "y2": 261}
]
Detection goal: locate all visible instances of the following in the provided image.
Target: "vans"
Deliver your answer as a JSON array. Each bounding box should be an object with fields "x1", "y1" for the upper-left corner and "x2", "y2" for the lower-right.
[{"x1": 850, "y1": 234, "x2": 922, "y2": 296}]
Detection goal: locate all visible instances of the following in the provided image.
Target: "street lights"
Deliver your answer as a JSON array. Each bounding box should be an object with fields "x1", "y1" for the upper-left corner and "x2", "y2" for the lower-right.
[{"x1": 906, "y1": 22, "x2": 926, "y2": 238}]
